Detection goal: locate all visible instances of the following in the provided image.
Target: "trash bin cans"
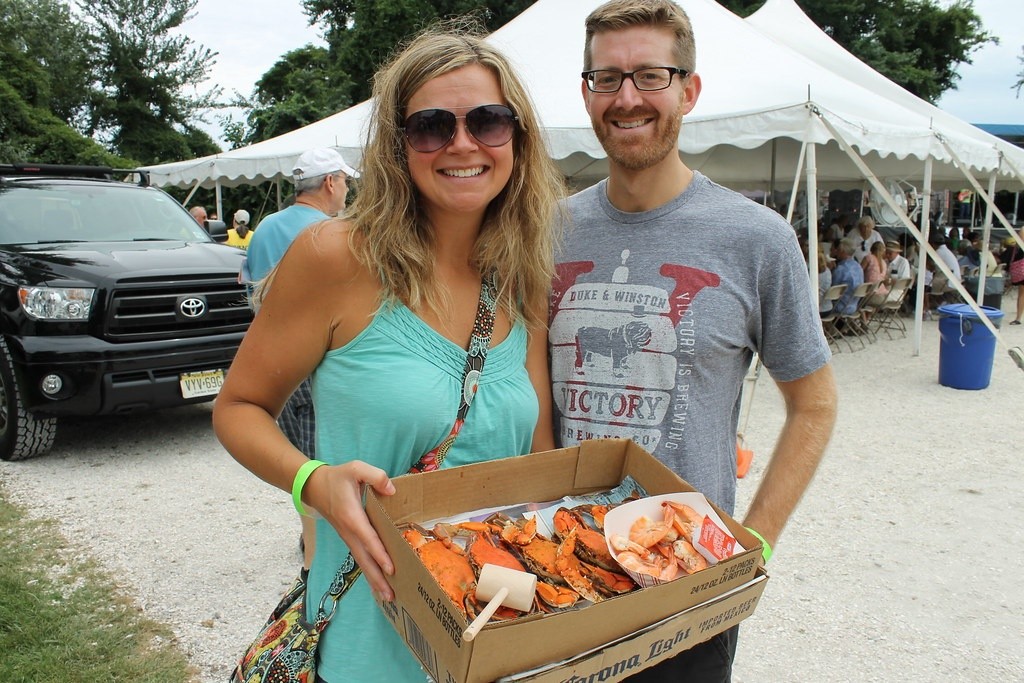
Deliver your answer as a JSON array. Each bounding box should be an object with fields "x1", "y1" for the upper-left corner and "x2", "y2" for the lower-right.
[
  {"x1": 937, "y1": 304, "x2": 1004, "y2": 390},
  {"x1": 964, "y1": 276, "x2": 1004, "y2": 310}
]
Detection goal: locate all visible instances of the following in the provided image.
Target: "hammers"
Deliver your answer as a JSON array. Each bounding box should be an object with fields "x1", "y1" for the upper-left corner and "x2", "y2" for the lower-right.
[{"x1": 462, "y1": 563, "x2": 537, "y2": 642}]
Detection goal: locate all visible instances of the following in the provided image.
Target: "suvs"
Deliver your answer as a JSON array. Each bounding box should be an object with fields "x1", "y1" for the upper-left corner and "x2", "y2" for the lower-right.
[{"x1": 0, "y1": 163, "x2": 254, "y2": 463}]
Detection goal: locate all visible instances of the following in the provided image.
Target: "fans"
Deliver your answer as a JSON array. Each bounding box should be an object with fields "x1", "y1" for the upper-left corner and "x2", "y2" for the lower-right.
[{"x1": 868, "y1": 175, "x2": 919, "y2": 227}]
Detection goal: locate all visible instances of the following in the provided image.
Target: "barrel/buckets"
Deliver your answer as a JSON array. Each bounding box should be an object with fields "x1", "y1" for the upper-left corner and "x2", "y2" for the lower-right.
[{"x1": 937, "y1": 304, "x2": 1004, "y2": 391}]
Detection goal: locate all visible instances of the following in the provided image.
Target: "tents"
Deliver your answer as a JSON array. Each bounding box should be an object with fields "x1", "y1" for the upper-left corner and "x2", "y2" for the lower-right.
[
  {"x1": 122, "y1": 0, "x2": 1001, "y2": 370},
  {"x1": 742, "y1": 0, "x2": 1024, "y2": 225}
]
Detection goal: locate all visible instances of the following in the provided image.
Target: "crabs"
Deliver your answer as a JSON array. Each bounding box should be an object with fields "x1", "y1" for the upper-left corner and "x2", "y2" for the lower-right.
[{"x1": 401, "y1": 490, "x2": 643, "y2": 624}]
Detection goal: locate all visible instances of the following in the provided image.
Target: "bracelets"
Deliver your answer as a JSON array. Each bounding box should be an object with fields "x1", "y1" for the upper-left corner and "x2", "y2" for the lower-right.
[
  {"x1": 291, "y1": 459, "x2": 329, "y2": 520},
  {"x1": 740, "y1": 525, "x2": 772, "y2": 566}
]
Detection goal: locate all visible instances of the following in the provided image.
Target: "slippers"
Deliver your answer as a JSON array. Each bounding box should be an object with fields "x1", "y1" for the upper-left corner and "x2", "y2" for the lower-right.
[{"x1": 1009, "y1": 319, "x2": 1021, "y2": 325}]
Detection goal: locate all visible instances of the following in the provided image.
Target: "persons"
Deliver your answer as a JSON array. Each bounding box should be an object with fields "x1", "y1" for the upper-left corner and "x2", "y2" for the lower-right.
[
  {"x1": 549, "y1": 1, "x2": 838, "y2": 683},
  {"x1": 236, "y1": 147, "x2": 360, "y2": 559},
  {"x1": 226, "y1": 209, "x2": 254, "y2": 251},
  {"x1": 212, "y1": 34, "x2": 556, "y2": 683},
  {"x1": 189, "y1": 206, "x2": 208, "y2": 228},
  {"x1": 795, "y1": 216, "x2": 1024, "y2": 371},
  {"x1": 209, "y1": 210, "x2": 216, "y2": 220}
]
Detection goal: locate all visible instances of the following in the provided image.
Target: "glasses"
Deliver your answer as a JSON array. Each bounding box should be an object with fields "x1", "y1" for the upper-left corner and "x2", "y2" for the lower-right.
[
  {"x1": 861, "y1": 241, "x2": 865, "y2": 252},
  {"x1": 398, "y1": 104, "x2": 521, "y2": 152},
  {"x1": 581, "y1": 65, "x2": 689, "y2": 93}
]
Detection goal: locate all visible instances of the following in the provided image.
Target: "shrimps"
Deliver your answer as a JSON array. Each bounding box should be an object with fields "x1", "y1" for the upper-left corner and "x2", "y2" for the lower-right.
[{"x1": 610, "y1": 502, "x2": 714, "y2": 581}]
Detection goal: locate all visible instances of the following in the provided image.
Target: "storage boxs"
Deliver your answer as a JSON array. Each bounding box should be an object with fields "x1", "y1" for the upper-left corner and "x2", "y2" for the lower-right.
[{"x1": 359, "y1": 437, "x2": 768, "y2": 683}]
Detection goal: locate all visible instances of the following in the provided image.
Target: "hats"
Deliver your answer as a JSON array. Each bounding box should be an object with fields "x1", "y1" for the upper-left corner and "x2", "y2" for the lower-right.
[
  {"x1": 234, "y1": 209, "x2": 250, "y2": 226},
  {"x1": 884, "y1": 241, "x2": 902, "y2": 253},
  {"x1": 932, "y1": 234, "x2": 945, "y2": 243},
  {"x1": 291, "y1": 152, "x2": 362, "y2": 180},
  {"x1": 966, "y1": 245, "x2": 981, "y2": 267}
]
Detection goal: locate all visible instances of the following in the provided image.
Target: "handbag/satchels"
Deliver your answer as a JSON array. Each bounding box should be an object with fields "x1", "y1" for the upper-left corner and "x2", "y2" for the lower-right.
[
  {"x1": 229, "y1": 575, "x2": 328, "y2": 683},
  {"x1": 1009, "y1": 258, "x2": 1024, "y2": 284}
]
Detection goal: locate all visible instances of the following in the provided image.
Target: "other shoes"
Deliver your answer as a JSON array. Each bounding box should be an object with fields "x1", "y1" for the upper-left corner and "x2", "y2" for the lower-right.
[
  {"x1": 848, "y1": 323, "x2": 867, "y2": 336},
  {"x1": 923, "y1": 312, "x2": 933, "y2": 322}
]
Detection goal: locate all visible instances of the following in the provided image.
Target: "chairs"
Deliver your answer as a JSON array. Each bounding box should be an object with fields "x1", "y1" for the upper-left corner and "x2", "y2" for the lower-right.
[{"x1": 819, "y1": 261, "x2": 1007, "y2": 353}]
